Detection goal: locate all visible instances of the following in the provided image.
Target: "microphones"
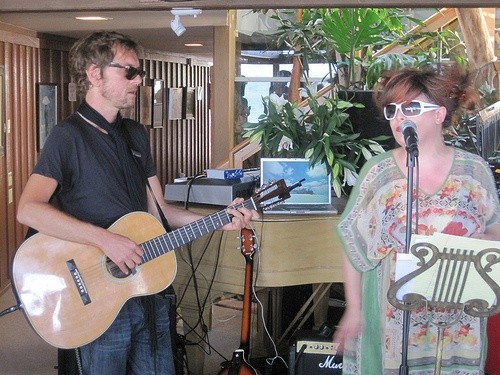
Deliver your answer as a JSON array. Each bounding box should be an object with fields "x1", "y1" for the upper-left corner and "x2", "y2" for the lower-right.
[{"x1": 399, "y1": 120, "x2": 419, "y2": 157}]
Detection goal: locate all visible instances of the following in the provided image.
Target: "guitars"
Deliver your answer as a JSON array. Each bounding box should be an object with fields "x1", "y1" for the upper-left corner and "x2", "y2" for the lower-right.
[
  {"x1": 0, "y1": 173, "x2": 308, "y2": 351},
  {"x1": 216, "y1": 228, "x2": 267, "y2": 375}
]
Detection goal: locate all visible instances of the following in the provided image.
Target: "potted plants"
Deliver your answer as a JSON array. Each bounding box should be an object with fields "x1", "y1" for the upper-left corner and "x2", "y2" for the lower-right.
[{"x1": 301, "y1": 7, "x2": 468, "y2": 198}]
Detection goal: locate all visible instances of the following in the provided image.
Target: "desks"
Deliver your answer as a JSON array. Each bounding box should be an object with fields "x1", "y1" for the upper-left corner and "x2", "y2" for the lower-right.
[{"x1": 166, "y1": 198, "x2": 346, "y2": 375}]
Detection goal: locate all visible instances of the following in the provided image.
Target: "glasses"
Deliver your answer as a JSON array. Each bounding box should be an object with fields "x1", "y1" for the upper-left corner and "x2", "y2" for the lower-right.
[
  {"x1": 107, "y1": 62, "x2": 147, "y2": 80},
  {"x1": 383, "y1": 100, "x2": 441, "y2": 120}
]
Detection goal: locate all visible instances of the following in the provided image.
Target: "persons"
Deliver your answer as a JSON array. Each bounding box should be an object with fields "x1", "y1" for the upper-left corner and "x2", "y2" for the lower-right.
[
  {"x1": 15, "y1": 30, "x2": 260, "y2": 375},
  {"x1": 332, "y1": 60, "x2": 500, "y2": 375}
]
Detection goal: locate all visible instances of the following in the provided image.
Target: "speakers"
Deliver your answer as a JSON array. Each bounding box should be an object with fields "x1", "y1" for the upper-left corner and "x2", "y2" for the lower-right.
[{"x1": 288, "y1": 329, "x2": 344, "y2": 375}]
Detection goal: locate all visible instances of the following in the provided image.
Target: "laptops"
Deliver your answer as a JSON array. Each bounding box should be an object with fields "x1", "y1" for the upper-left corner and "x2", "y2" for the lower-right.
[{"x1": 260, "y1": 158, "x2": 338, "y2": 215}]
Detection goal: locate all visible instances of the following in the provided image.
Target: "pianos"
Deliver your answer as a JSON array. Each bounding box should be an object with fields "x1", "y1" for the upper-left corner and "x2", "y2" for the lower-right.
[{"x1": 163, "y1": 165, "x2": 261, "y2": 205}]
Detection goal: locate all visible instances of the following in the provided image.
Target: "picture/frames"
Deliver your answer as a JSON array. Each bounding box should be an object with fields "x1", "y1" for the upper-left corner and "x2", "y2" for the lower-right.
[
  {"x1": 168, "y1": 87, "x2": 183, "y2": 121},
  {"x1": 138, "y1": 85, "x2": 153, "y2": 126},
  {"x1": 184, "y1": 86, "x2": 197, "y2": 120},
  {"x1": 35, "y1": 82, "x2": 59, "y2": 153},
  {"x1": 151, "y1": 79, "x2": 165, "y2": 129}
]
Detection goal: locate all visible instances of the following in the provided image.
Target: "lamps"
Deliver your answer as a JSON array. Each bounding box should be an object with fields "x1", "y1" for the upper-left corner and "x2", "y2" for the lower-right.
[{"x1": 170, "y1": 14, "x2": 186, "y2": 37}]
